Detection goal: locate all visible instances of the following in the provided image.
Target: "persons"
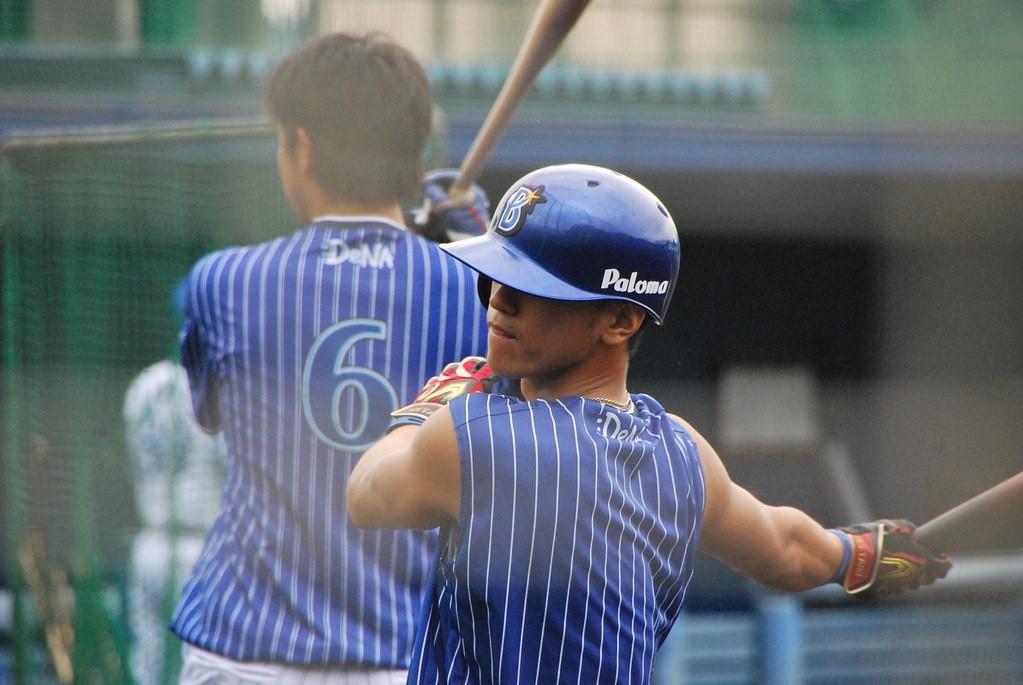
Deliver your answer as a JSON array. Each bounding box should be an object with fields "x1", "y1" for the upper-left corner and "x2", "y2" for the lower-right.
[
  {"x1": 167, "y1": 36, "x2": 493, "y2": 684},
  {"x1": 347, "y1": 163, "x2": 953, "y2": 685},
  {"x1": 121, "y1": 358, "x2": 229, "y2": 685}
]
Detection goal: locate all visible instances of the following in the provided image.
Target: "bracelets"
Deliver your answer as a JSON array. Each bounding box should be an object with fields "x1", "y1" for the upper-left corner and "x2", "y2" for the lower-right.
[
  {"x1": 385, "y1": 417, "x2": 422, "y2": 432},
  {"x1": 826, "y1": 529, "x2": 852, "y2": 583},
  {"x1": 590, "y1": 392, "x2": 633, "y2": 411}
]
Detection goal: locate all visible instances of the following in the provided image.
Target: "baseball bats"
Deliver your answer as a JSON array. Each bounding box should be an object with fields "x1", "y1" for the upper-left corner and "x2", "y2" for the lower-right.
[
  {"x1": 449, "y1": 0, "x2": 595, "y2": 200},
  {"x1": 909, "y1": 471, "x2": 1023, "y2": 557}
]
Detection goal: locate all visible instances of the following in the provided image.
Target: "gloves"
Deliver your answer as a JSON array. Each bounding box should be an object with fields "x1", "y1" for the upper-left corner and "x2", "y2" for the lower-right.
[
  {"x1": 835, "y1": 518, "x2": 954, "y2": 603},
  {"x1": 385, "y1": 356, "x2": 527, "y2": 435},
  {"x1": 408, "y1": 169, "x2": 492, "y2": 244}
]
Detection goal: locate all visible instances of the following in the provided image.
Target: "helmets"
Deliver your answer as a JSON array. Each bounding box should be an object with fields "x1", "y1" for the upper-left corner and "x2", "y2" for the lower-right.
[{"x1": 438, "y1": 164, "x2": 681, "y2": 326}]
